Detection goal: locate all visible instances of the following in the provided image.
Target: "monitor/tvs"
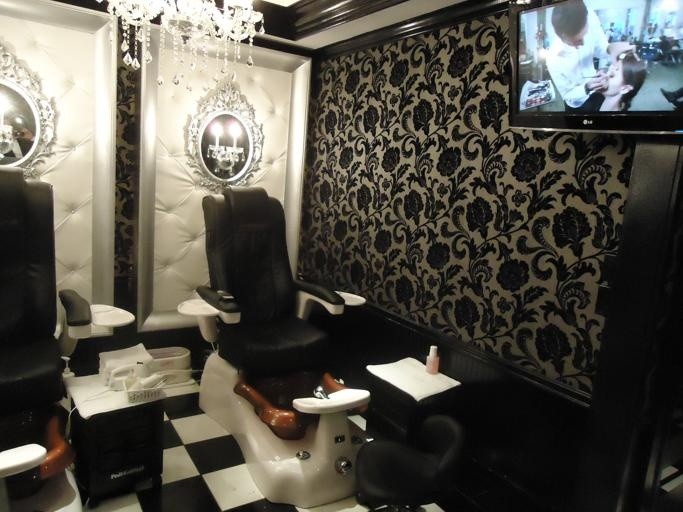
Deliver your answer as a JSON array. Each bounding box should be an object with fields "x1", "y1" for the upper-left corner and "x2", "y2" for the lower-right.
[{"x1": 508, "y1": 0, "x2": 683, "y2": 135}]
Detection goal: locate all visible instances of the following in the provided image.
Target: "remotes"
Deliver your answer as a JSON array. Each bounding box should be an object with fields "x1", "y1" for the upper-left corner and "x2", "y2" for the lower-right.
[{"x1": 216, "y1": 289, "x2": 235, "y2": 302}]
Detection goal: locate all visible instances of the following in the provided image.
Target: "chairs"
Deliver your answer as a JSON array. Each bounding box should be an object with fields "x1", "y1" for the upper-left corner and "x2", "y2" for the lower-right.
[
  {"x1": 176, "y1": 185, "x2": 460, "y2": 511},
  {"x1": 0, "y1": 166, "x2": 199, "y2": 512}
]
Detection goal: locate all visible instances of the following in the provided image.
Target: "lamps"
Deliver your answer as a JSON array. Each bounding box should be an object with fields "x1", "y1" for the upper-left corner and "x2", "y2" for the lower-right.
[
  {"x1": 0, "y1": 95, "x2": 23, "y2": 160},
  {"x1": 97, "y1": 0, "x2": 265, "y2": 92},
  {"x1": 208, "y1": 121, "x2": 246, "y2": 176}
]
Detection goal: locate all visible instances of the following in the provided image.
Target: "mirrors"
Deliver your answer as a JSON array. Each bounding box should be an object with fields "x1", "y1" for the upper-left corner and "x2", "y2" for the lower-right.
[
  {"x1": 0, "y1": 36, "x2": 59, "y2": 180},
  {"x1": 185, "y1": 72, "x2": 264, "y2": 191}
]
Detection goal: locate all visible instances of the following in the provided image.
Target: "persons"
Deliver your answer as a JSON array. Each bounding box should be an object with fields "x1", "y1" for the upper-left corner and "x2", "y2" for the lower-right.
[
  {"x1": 599, "y1": 53, "x2": 645, "y2": 111},
  {"x1": 544, "y1": 0, "x2": 640, "y2": 113}
]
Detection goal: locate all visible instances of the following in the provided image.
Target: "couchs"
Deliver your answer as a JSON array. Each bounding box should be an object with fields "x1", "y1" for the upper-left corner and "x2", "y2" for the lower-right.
[{"x1": 354, "y1": 414, "x2": 471, "y2": 512}]
